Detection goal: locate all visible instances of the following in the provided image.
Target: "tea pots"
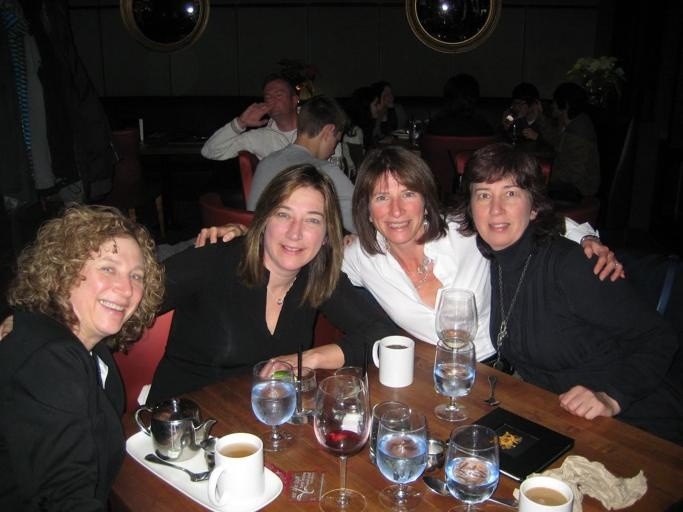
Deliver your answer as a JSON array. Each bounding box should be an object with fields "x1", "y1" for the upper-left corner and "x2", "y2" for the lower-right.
[{"x1": 135, "y1": 398, "x2": 216, "y2": 463}]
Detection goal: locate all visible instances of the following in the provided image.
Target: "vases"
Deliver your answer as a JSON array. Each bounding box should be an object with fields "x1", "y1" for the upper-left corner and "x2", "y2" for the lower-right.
[
  {"x1": 587, "y1": 83, "x2": 599, "y2": 106},
  {"x1": 597, "y1": 85, "x2": 611, "y2": 108}
]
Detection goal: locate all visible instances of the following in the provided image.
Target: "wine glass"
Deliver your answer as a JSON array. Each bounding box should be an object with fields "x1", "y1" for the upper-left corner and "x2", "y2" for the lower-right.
[
  {"x1": 314, "y1": 375, "x2": 372, "y2": 512},
  {"x1": 445, "y1": 425, "x2": 500, "y2": 512},
  {"x1": 251, "y1": 361, "x2": 297, "y2": 451},
  {"x1": 434, "y1": 338, "x2": 477, "y2": 422},
  {"x1": 434, "y1": 289, "x2": 478, "y2": 352},
  {"x1": 376, "y1": 409, "x2": 427, "y2": 511}
]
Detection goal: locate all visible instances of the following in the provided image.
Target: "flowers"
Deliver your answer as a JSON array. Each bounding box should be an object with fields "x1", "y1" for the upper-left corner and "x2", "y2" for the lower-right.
[
  {"x1": 586, "y1": 55, "x2": 627, "y2": 97},
  {"x1": 565, "y1": 54, "x2": 599, "y2": 86}
]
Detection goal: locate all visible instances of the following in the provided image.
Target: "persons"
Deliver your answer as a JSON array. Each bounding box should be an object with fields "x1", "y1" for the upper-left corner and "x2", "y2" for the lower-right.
[
  {"x1": 195, "y1": 145, "x2": 624, "y2": 377},
  {"x1": 0, "y1": 198, "x2": 167, "y2": 511},
  {"x1": 447, "y1": 143, "x2": 683, "y2": 446},
  {"x1": 201, "y1": 71, "x2": 398, "y2": 235},
  {"x1": 427, "y1": 73, "x2": 599, "y2": 206},
  {"x1": 1, "y1": 163, "x2": 400, "y2": 406}
]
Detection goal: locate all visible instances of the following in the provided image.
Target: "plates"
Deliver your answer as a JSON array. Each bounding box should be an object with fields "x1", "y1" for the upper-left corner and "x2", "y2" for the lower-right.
[{"x1": 126, "y1": 426, "x2": 284, "y2": 512}]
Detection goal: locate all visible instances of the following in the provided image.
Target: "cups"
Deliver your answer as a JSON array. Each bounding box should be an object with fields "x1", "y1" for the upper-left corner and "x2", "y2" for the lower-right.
[
  {"x1": 334, "y1": 365, "x2": 370, "y2": 387},
  {"x1": 208, "y1": 432, "x2": 264, "y2": 506},
  {"x1": 373, "y1": 336, "x2": 415, "y2": 388},
  {"x1": 518, "y1": 477, "x2": 573, "y2": 512},
  {"x1": 369, "y1": 400, "x2": 410, "y2": 465},
  {"x1": 287, "y1": 366, "x2": 318, "y2": 425}
]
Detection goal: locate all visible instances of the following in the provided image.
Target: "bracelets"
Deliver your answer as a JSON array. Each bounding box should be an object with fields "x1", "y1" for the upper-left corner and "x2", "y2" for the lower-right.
[{"x1": 580, "y1": 235, "x2": 602, "y2": 243}]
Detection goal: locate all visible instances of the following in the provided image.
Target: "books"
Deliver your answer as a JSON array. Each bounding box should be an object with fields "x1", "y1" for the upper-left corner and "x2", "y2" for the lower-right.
[{"x1": 449, "y1": 405, "x2": 574, "y2": 485}]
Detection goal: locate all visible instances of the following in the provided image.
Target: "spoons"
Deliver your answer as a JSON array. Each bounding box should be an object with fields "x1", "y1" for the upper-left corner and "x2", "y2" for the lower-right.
[
  {"x1": 422, "y1": 476, "x2": 518, "y2": 509},
  {"x1": 482, "y1": 375, "x2": 501, "y2": 406},
  {"x1": 144, "y1": 453, "x2": 210, "y2": 482}
]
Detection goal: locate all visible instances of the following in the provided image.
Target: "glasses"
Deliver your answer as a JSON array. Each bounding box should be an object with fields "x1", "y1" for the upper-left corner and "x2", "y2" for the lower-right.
[{"x1": 512, "y1": 101, "x2": 528, "y2": 105}]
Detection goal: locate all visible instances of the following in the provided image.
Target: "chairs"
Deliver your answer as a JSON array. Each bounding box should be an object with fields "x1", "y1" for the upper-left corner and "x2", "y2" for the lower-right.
[
  {"x1": 613, "y1": 252, "x2": 677, "y2": 316},
  {"x1": 111, "y1": 308, "x2": 177, "y2": 412}
]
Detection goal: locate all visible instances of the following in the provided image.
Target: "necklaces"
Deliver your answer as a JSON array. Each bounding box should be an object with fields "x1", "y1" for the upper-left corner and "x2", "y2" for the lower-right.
[
  {"x1": 267, "y1": 283, "x2": 294, "y2": 305},
  {"x1": 406, "y1": 258, "x2": 434, "y2": 286},
  {"x1": 493, "y1": 249, "x2": 534, "y2": 375}
]
